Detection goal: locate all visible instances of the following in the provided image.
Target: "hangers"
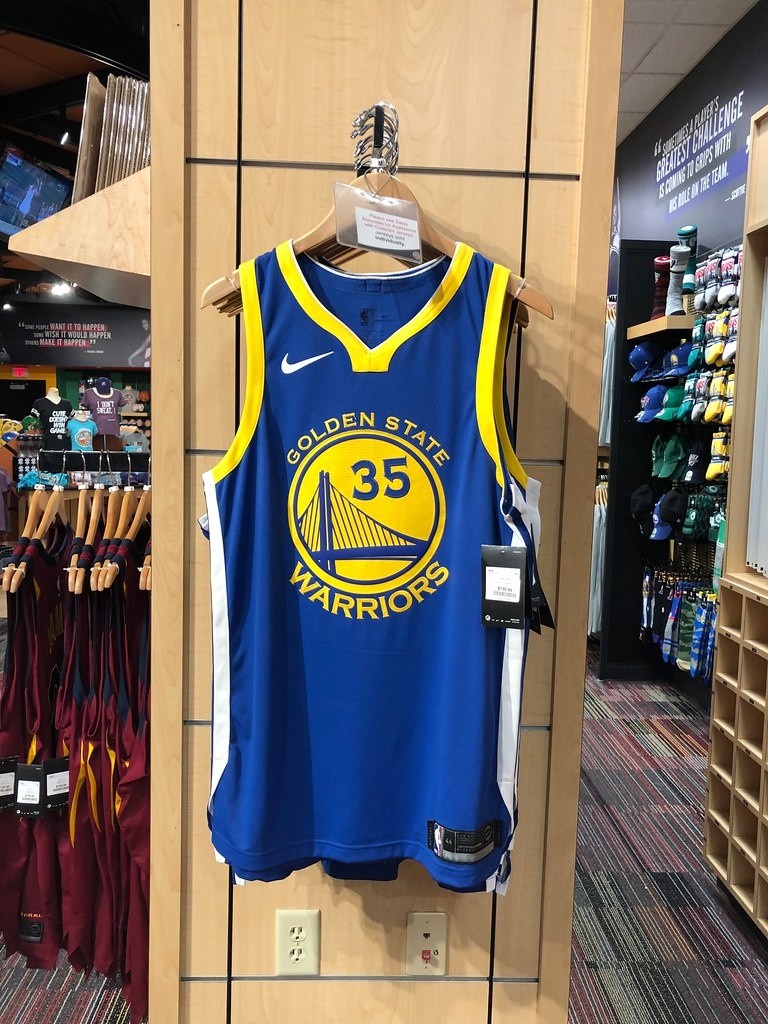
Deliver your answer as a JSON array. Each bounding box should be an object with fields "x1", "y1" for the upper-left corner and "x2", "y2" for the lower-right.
[
  {"x1": 3, "y1": 449, "x2": 151, "y2": 594},
  {"x1": 203, "y1": 102, "x2": 553, "y2": 331}
]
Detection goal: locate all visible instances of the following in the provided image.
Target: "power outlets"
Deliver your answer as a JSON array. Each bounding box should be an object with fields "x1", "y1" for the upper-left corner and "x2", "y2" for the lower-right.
[{"x1": 275, "y1": 909, "x2": 322, "y2": 976}]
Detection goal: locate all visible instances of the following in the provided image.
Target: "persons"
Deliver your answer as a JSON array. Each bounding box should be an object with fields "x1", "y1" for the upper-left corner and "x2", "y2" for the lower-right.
[
  {"x1": 31, "y1": 387, "x2": 75, "y2": 451},
  {"x1": 13, "y1": 177, "x2": 61, "y2": 229},
  {"x1": 83, "y1": 378, "x2": 124, "y2": 436},
  {"x1": 66, "y1": 411, "x2": 98, "y2": 451},
  {"x1": 119, "y1": 385, "x2": 140, "y2": 412}
]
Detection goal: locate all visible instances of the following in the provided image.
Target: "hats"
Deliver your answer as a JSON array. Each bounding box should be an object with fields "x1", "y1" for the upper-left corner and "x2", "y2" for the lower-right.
[
  {"x1": 634, "y1": 394, "x2": 645, "y2": 418},
  {"x1": 631, "y1": 484, "x2": 655, "y2": 535},
  {"x1": 649, "y1": 494, "x2": 672, "y2": 540},
  {"x1": 660, "y1": 487, "x2": 688, "y2": 536},
  {"x1": 662, "y1": 342, "x2": 695, "y2": 377},
  {"x1": 651, "y1": 432, "x2": 712, "y2": 484},
  {"x1": 653, "y1": 385, "x2": 685, "y2": 421},
  {"x1": 629, "y1": 342, "x2": 656, "y2": 382},
  {"x1": 95, "y1": 377, "x2": 111, "y2": 395},
  {"x1": 637, "y1": 384, "x2": 670, "y2": 423},
  {"x1": 652, "y1": 352, "x2": 673, "y2": 378}
]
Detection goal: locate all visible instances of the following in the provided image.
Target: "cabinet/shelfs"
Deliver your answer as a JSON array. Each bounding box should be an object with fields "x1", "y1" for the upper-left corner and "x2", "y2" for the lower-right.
[{"x1": 704, "y1": 577, "x2": 768, "y2": 941}]
